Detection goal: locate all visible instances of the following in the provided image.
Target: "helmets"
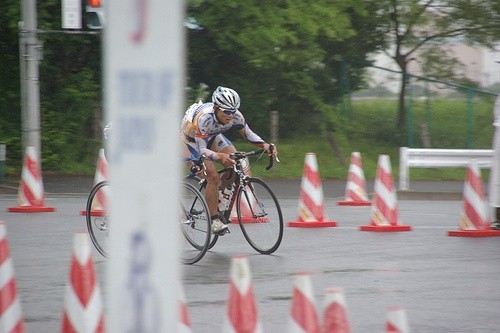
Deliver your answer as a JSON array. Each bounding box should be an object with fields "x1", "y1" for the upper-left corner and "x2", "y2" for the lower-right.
[{"x1": 211, "y1": 86, "x2": 241, "y2": 109}]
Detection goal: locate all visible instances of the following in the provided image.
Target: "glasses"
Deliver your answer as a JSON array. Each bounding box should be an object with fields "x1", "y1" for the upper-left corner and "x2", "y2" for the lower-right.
[{"x1": 215, "y1": 106, "x2": 236, "y2": 115}]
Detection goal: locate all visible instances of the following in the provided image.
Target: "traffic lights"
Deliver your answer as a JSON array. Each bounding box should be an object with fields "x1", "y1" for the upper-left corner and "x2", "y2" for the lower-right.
[{"x1": 85, "y1": 0, "x2": 103, "y2": 30}]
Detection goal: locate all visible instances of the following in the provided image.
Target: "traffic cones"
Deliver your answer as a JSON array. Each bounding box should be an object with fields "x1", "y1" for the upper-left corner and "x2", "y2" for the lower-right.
[
  {"x1": 0, "y1": 218, "x2": 25, "y2": 332},
  {"x1": 385, "y1": 304, "x2": 409, "y2": 332},
  {"x1": 336, "y1": 151, "x2": 372, "y2": 206},
  {"x1": 180, "y1": 296, "x2": 192, "y2": 332},
  {"x1": 288, "y1": 272, "x2": 318, "y2": 333},
  {"x1": 7, "y1": 146, "x2": 55, "y2": 212},
  {"x1": 79, "y1": 148, "x2": 109, "y2": 216},
  {"x1": 58, "y1": 232, "x2": 105, "y2": 333},
  {"x1": 320, "y1": 287, "x2": 347, "y2": 333},
  {"x1": 288, "y1": 153, "x2": 336, "y2": 227},
  {"x1": 225, "y1": 255, "x2": 261, "y2": 332},
  {"x1": 358, "y1": 153, "x2": 413, "y2": 232},
  {"x1": 447, "y1": 163, "x2": 499, "y2": 236}
]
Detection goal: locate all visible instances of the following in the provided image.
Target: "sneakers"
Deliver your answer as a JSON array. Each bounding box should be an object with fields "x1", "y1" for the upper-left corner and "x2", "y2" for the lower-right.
[
  {"x1": 218, "y1": 190, "x2": 230, "y2": 210},
  {"x1": 211, "y1": 219, "x2": 228, "y2": 232}
]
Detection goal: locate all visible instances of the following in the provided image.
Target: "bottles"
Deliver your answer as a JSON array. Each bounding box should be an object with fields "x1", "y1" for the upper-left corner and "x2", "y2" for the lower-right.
[
  {"x1": 222, "y1": 185, "x2": 233, "y2": 210},
  {"x1": 217, "y1": 188, "x2": 225, "y2": 211}
]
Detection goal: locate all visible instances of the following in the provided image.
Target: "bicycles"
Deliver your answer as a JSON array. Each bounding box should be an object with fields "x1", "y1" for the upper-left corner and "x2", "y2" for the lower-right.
[
  {"x1": 185, "y1": 143, "x2": 284, "y2": 254},
  {"x1": 85, "y1": 154, "x2": 212, "y2": 266}
]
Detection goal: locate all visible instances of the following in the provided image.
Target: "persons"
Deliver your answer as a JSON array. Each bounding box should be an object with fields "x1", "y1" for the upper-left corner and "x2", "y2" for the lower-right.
[{"x1": 97, "y1": 86, "x2": 277, "y2": 238}]
